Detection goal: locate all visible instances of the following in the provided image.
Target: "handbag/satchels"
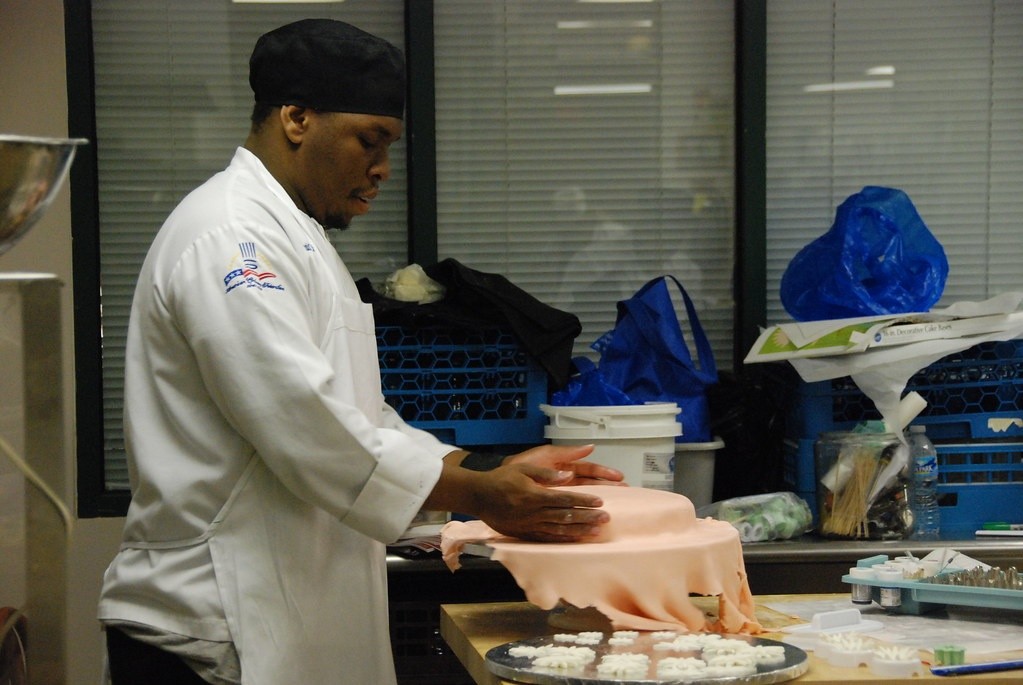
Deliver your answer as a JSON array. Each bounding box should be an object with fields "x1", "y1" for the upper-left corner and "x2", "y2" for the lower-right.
[
  {"x1": 780, "y1": 184, "x2": 949, "y2": 321},
  {"x1": 591, "y1": 275, "x2": 717, "y2": 398}
]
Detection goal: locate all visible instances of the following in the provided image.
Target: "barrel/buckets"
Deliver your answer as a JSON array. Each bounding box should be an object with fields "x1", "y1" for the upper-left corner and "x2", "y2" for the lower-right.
[{"x1": 540, "y1": 401, "x2": 684, "y2": 494}]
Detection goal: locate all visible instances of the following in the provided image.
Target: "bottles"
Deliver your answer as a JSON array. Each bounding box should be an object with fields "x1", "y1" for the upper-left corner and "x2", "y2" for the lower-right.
[
  {"x1": 905, "y1": 425, "x2": 941, "y2": 538},
  {"x1": 813, "y1": 430, "x2": 917, "y2": 540}
]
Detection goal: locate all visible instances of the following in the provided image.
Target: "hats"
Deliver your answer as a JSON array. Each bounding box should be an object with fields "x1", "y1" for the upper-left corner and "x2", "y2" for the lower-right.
[{"x1": 249, "y1": 18, "x2": 406, "y2": 121}]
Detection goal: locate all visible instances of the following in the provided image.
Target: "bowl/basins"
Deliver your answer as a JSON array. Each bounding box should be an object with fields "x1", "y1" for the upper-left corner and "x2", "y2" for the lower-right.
[{"x1": 0, "y1": 133, "x2": 89, "y2": 260}]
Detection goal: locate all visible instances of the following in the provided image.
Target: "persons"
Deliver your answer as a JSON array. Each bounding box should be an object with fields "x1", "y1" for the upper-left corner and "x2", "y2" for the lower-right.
[{"x1": 97, "y1": 17, "x2": 629, "y2": 684}]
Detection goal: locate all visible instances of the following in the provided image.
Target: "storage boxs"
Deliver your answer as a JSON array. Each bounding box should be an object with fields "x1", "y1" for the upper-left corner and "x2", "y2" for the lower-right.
[{"x1": 375, "y1": 274, "x2": 1023, "y2": 542}]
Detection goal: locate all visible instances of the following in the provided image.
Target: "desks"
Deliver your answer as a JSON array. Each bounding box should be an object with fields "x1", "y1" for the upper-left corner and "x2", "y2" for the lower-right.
[{"x1": 439, "y1": 586, "x2": 1023, "y2": 685}]
